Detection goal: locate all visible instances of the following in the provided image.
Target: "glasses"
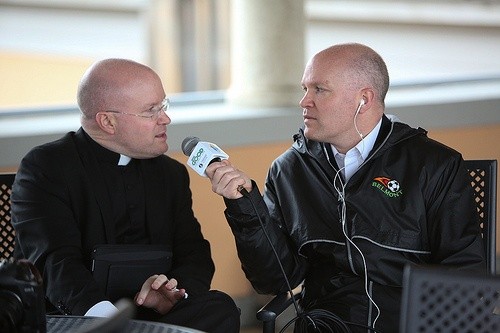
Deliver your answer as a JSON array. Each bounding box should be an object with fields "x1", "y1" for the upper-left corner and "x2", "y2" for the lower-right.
[{"x1": 106, "y1": 98, "x2": 169, "y2": 119}]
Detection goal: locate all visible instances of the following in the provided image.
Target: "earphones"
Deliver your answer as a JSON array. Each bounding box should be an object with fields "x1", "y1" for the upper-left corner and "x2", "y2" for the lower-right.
[{"x1": 360, "y1": 99, "x2": 365, "y2": 105}]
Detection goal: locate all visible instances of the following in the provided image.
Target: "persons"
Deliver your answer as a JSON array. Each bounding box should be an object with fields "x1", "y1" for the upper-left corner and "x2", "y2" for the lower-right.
[
  {"x1": 207, "y1": 42, "x2": 484, "y2": 333},
  {"x1": 10, "y1": 58, "x2": 241, "y2": 333}
]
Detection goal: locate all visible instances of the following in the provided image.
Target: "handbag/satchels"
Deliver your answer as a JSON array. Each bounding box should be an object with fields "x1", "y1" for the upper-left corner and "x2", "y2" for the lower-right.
[{"x1": 89, "y1": 243, "x2": 173, "y2": 303}]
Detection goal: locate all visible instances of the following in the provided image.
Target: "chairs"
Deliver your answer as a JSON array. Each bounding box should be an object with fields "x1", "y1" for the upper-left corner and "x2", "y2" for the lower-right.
[{"x1": 258, "y1": 159, "x2": 500, "y2": 333}]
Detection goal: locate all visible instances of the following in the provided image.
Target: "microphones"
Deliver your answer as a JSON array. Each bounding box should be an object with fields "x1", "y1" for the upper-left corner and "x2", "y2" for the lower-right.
[{"x1": 181, "y1": 136, "x2": 253, "y2": 198}]
